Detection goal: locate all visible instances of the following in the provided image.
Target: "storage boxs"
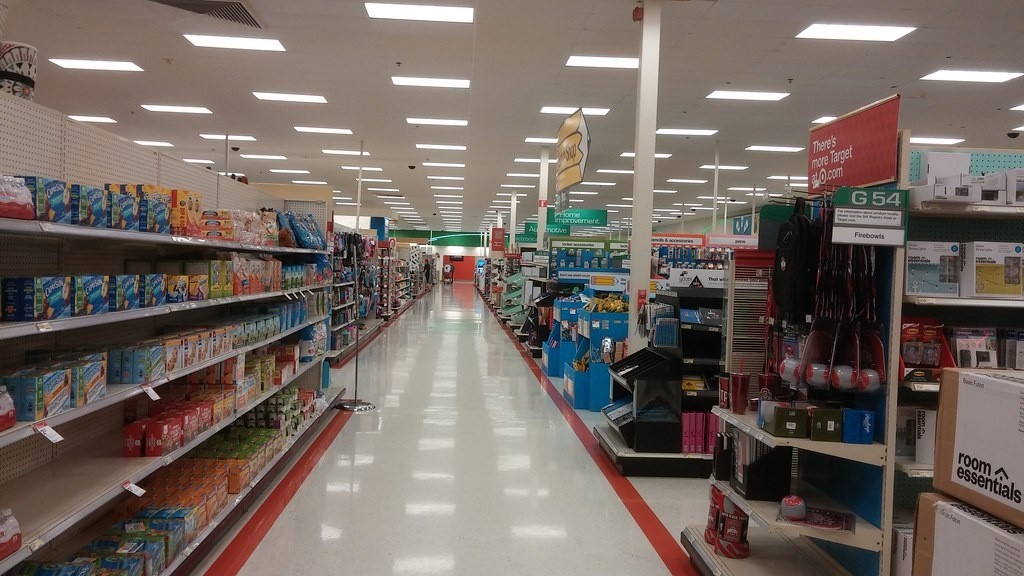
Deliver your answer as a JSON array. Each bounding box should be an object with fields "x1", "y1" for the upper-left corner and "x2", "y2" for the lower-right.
[
  {"x1": 933, "y1": 366, "x2": 1024, "y2": 532},
  {"x1": 911, "y1": 493, "x2": 1023, "y2": 576},
  {"x1": 2, "y1": 171, "x2": 330, "y2": 575},
  {"x1": 893, "y1": 152, "x2": 1023, "y2": 575}
]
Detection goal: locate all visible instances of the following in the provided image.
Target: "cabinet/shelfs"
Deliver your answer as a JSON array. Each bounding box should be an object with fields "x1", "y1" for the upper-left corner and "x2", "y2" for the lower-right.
[
  {"x1": 0, "y1": 91, "x2": 337, "y2": 576},
  {"x1": 330, "y1": 222, "x2": 436, "y2": 368},
  {"x1": 476, "y1": 235, "x2": 892, "y2": 576},
  {"x1": 892, "y1": 201, "x2": 1024, "y2": 476}
]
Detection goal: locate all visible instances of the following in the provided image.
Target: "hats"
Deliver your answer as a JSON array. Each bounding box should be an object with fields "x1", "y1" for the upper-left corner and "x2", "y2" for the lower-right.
[{"x1": 425, "y1": 259, "x2": 428, "y2": 262}]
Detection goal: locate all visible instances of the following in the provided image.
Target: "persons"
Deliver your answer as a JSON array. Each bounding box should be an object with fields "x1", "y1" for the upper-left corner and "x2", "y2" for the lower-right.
[
  {"x1": 449, "y1": 262, "x2": 455, "y2": 284},
  {"x1": 423, "y1": 259, "x2": 431, "y2": 292}
]
